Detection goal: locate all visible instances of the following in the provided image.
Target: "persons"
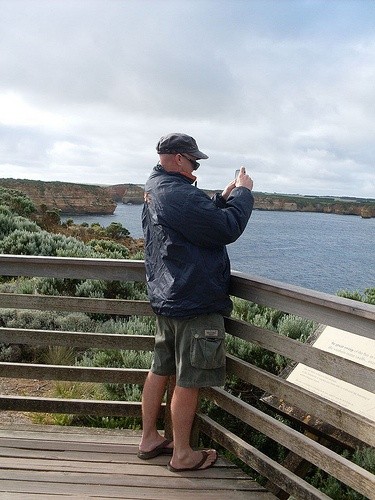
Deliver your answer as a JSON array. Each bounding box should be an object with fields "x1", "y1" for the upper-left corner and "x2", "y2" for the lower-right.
[{"x1": 136, "y1": 132, "x2": 252, "y2": 471}]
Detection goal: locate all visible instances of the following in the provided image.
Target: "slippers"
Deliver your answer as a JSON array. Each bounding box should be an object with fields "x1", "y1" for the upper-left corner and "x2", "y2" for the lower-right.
[
  {"x1": 139, "y1": 438, "x2": 172, "y2": 459},
  {"x1": 167, "y1": 449, "x2": 218, "y2": 471}
]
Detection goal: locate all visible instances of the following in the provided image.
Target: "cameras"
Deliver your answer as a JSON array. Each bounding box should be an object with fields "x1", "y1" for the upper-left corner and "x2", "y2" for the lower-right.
[{"x1": 235, "y1": 170, "x2": 245, "y2": 179}]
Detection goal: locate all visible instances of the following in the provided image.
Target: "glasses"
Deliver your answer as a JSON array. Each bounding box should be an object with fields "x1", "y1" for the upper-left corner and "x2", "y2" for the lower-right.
[{"x1": 179, "y1": 153, "x2": 200, "y2": 171}]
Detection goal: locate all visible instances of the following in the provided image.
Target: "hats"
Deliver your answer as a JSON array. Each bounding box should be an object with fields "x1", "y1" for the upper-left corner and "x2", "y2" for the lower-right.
[{"x1": 157, "y1": 133, "x2": 209, "y2": 160}]
